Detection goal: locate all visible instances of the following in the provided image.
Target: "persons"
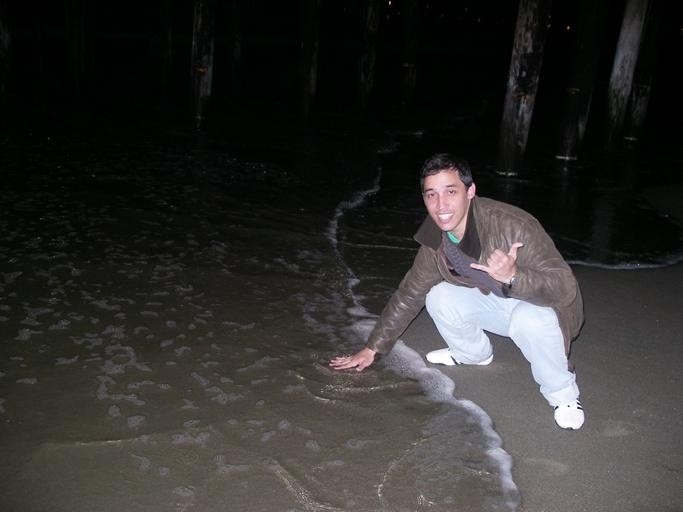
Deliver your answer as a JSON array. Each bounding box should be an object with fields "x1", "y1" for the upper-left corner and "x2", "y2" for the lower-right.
[{"x1": 330, "y1": 153, "x2": 585, "y2": 429}]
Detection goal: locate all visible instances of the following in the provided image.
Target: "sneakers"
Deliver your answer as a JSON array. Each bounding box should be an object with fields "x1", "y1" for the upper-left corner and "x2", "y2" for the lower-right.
[
  {"x1": 425, "y1": 346, "x2": 495, "y2": 366},
  {"x1": 553, "y1": 397, "x2": 586, "y2": 432}
]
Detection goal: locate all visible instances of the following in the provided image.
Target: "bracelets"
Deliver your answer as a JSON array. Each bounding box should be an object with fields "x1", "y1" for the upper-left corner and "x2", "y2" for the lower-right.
[{"x1": 509, "y1": 275, "x2": 516, "y2": 288}]
